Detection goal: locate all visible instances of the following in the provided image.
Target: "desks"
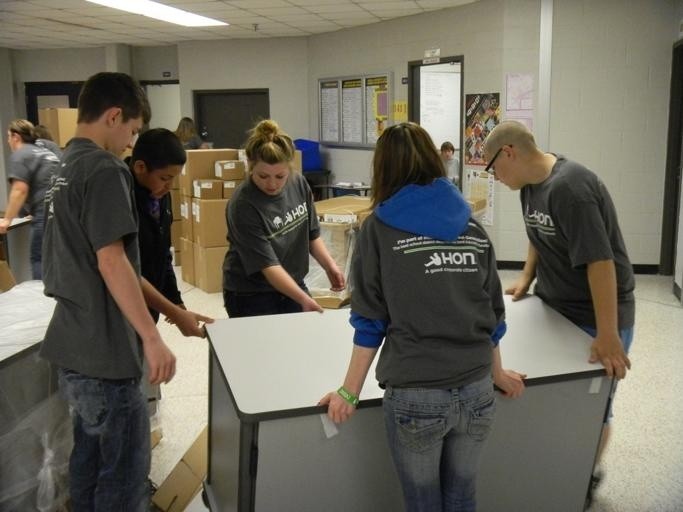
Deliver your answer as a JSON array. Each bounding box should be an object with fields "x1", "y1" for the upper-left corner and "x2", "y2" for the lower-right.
[
  {"x1": 0, "y1": 272, "x2": 75, "y2": 512},
  {"x1": 202, "y1": 287, "x2": 618, "y2": 511}
]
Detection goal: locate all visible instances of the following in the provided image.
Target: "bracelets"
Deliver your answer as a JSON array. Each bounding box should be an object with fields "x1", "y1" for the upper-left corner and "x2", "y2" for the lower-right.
[{"x1": 337, "y1": 387, "x2": 359, "y2": 406}]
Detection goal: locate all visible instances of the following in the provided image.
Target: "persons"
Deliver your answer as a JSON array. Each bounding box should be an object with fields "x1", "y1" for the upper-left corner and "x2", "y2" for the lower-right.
[
  {"x1": 41, "y1": 71, "x2": 175, "y2": 512},
  {"x1": 123, "y1": 128, "x2": 214, "y2": 493},
  {"x1": 482, "y1": 120, "x2": 634, "y2": 512},
  {"x1": 36, "y1": 124, "x2": 62, "y2": 161},
  {"x1": 318, "y1": 121, "x2": 526, "y2": 511},
  {"x1": 0, "y1": 119, "x2": 59, "y2": 280},
  {"x1": 174, "y1": 118, "x2": 208, "y2": 149},
  {"x1": 222, "y1": 120, "x2": 345, "y2": 317},
  {"x1": 437, "y1": 140, "x2": 460, "y2": 182}
]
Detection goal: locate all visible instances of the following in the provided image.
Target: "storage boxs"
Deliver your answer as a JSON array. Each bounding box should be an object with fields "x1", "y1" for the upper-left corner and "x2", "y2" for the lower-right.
[
  {"x1": 121, "y1": 140, "x2": 377, "y2": 295},
  {"x1": 36, "y1": 106, "x2": 81, "y2": 150}
]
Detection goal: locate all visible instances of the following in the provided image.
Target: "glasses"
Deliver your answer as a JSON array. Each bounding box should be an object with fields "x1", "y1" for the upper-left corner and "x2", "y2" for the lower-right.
[{"x1": 484, "y1": 142, "x2": 514, "y2": 177}]
